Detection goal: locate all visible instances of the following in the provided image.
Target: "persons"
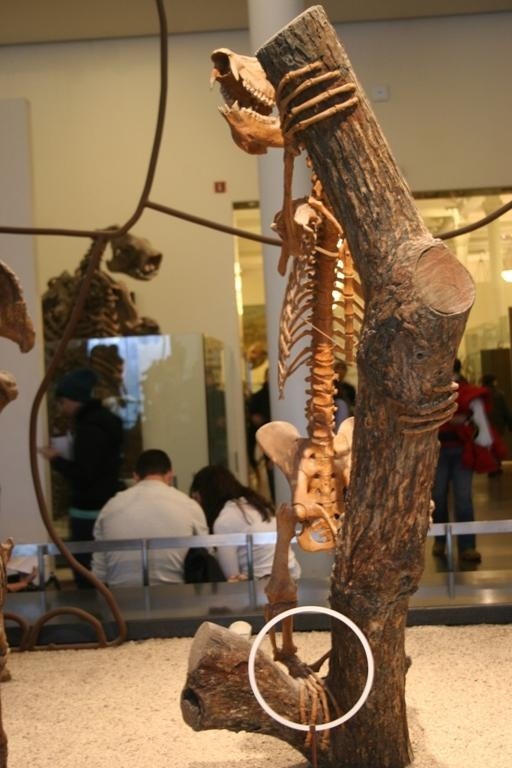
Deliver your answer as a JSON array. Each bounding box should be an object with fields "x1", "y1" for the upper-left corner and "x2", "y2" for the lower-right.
[
  {"x1": 334, "y1": 363, "x2": 355, "y2": 408},
  {"x1": 431, "y1": 359, "x2": 492, "y2": 560},
  {"x1": 37, "y1": 368, "x2": 301, "y2": 590},
  {"x1": 478, "y1": 374, "x2": 512, "y2": 477}
]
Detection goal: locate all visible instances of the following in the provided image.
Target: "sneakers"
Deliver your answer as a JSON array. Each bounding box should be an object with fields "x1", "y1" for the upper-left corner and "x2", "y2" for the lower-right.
[
  {"x1": 432, "y1": 542, "x2": 445, "y2": 554},
  {"x1": 459, "y1": 548, "x2": 481, "y2": 560}
]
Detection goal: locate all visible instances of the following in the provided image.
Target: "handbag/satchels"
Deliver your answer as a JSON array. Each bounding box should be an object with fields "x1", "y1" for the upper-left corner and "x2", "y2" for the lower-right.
[
  {"x1": 183, "y1": 548, "x2": 226, "y2": 583},
  {"x1": 462, "y1": 437, "x2": 498, "y2": 473}
]
